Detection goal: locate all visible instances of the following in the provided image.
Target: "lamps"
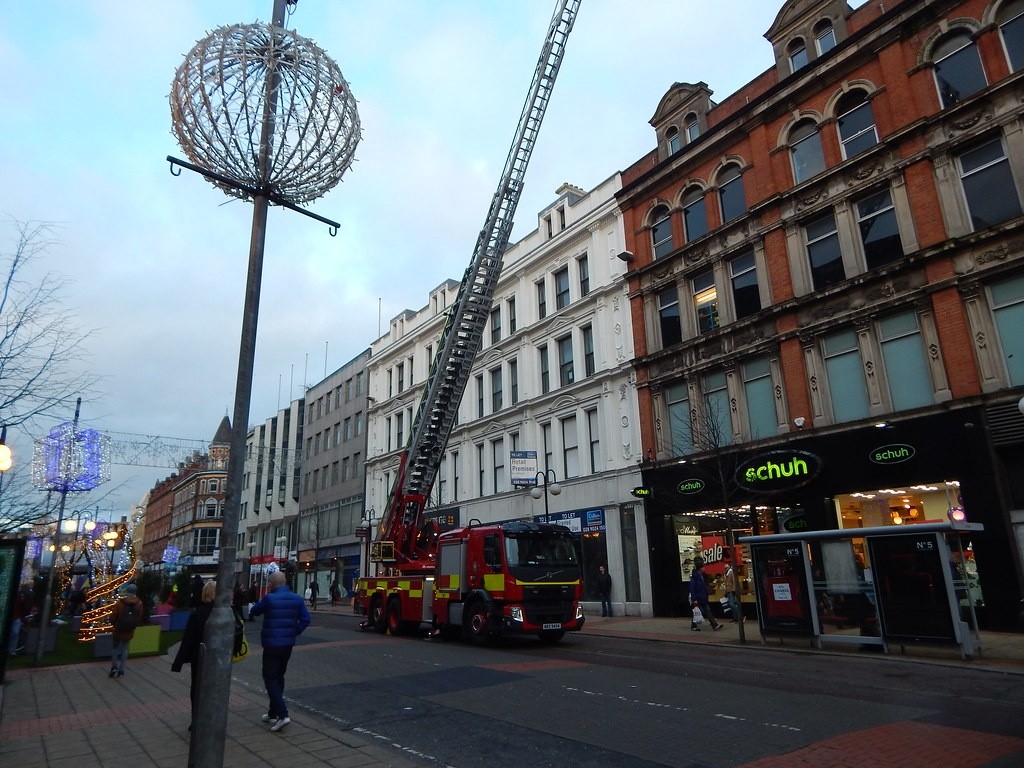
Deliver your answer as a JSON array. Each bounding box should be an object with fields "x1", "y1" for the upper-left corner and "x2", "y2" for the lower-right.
[
  {"x1": 617, "y1": 251, "x2": 633, "y2": 261},
  {"x1": 366, "y1": 396, "x2": 376, "y2": 401}
]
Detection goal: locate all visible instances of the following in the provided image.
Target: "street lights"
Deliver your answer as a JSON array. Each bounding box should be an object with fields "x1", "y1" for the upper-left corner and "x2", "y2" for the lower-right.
[
  {"x1": 275, "y1": 536, "x2": 287, "y2": 571},
  {"x1": 359, "y1": 508, "x2": 379, "y2": 578},
  {"x1": 531, "y1": 468, "x2": 561, "y2": 524},
  {"x1": 104, "y1": 531, "x2": 118, "y2": 567},
  {"x1": 247, "y1": 541, "x2": 258, "y2": 589},
  {"x1": 63, "y1": 509, "x2": 96, "y2": 612}
]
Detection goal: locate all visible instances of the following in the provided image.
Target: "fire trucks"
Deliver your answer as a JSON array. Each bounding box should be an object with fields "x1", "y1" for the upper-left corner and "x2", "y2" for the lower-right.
[{"x1": 351, "y1": 0, "x2": 589, "y2": 647}]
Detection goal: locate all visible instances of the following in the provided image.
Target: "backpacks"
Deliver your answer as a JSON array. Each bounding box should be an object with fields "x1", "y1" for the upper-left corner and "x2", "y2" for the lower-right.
[{"x1": 115, "y1": 599, "x2": 142, "y2": 632}]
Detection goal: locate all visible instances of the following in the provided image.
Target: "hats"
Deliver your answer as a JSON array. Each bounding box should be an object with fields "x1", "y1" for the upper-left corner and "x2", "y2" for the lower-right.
[{"x1": 126, "y1": 584, "x2": 137, "y2": 597}]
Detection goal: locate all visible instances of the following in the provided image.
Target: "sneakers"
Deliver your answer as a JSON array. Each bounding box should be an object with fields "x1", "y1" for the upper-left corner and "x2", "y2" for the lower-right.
[
  {"x1": 261, "y1": 713, "x2": 277, "y2": 723},
  {"x1": 270, "y1": 717, "x2": 291, "y2": 732}
]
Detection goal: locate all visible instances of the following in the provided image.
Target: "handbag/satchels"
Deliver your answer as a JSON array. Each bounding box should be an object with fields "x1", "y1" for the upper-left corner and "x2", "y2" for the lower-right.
[
  {"x1": 248, "y1": 602, "x2": 256, "y2": 614},
  {"x1": 692, "y1": 606, "x2": 705, "y2": 624},
  {"x1": 720, "y1": 597, "x2": 731, "y2": 612},
  {"x1": 327, "y1": 595, "x2": 333, "y2": 605}
]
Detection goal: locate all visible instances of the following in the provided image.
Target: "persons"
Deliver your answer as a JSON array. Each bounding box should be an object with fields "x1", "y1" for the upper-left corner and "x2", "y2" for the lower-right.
[
  {"x1": 598, "y1": 565, "x2": 613, "y2": 617},
  {"x1": 689, "y1": 563, "x2": 724, "y2": 631},
  {"x1": 141, "y1": 586, "x2": 155, "y2": 625},
  {"x1": 724, "y1": 562, "x2": 746, "y2": 624},
  {"x1": 109, "y1": 584, "x2": 143, "y2": 677},
  {"x1": 9, "y1": 584, "x2": 85, "y2": 654},
  {"x1": 310, "y1": 578, "x2": 341, "y2": 610},
  {"x1": 251, "y1": 571, "x2": 311, "y2": 731},
  {"x1": 172, "y1": 582, "x2": 243, "y2": 732},
  {"x1": 812, "y1": 568, "x2": 881, "y2": 638}
]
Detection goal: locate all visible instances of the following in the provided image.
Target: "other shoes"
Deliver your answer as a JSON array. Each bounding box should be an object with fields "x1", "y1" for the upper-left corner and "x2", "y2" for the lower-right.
[
  {"x1": 108, "y1": 666, "x2": 117, "y2": 678},
  {"x1": 743, "y1": 617, "x2": 746, "y2": 623},
  {"x1": 692, "y1": 627, "x2": 700, "y2": 631},
  {"x1": 118, "y1": 670, "x2": 124, "y2": 678},
  {"x1": 713, "y1": 624, "x2": 723, "y2": 631},
  {"x1": 10, "y1": 652, "x2": 17, "y2": 657},
  {"x1": 729, "y1": 620, "x2": 738, "y2": 624}
]
